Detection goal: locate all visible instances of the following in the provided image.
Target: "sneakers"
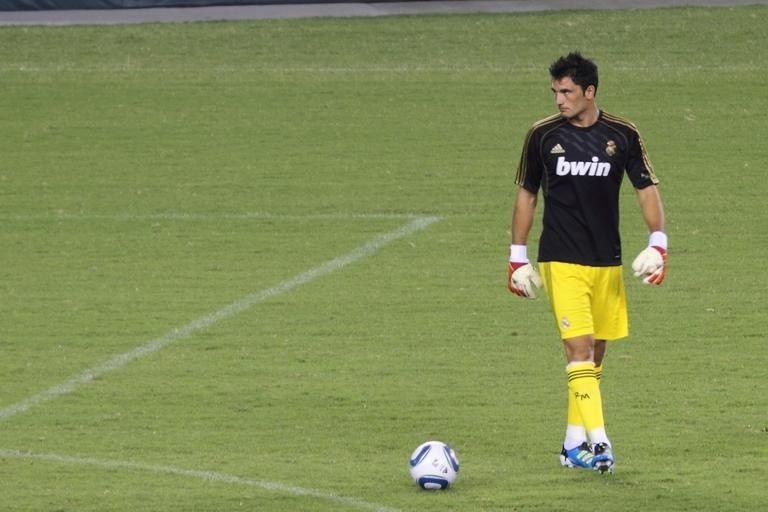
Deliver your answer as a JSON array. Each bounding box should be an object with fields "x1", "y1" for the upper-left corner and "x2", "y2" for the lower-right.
[
  {"x1": 559, "y1": 442, "x2": 608, "y2": 469},
  {"x1": 590, "y1": 442, "x2": 615, "y2": 476}
]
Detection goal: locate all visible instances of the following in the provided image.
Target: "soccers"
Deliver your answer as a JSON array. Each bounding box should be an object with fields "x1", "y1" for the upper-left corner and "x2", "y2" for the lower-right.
[{"x1": 409, "y1": 441, "x2": 459, "y2": 490}]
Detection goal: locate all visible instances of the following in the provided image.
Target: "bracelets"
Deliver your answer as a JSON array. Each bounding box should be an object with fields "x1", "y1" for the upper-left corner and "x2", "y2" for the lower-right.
[
  {"x1": 508, "y1": 244, "x2": 527, "y2": 260},
  {"x1": 646, "y1": 230, "x2": 667, "y2": 249}
]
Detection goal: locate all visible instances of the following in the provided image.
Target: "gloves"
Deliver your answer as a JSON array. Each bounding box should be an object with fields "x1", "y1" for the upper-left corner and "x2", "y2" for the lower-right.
[
  {"x1": 631, "y1": 231, "x2": 668, "y2": 287},
  {"x1": 507, "y1": 244, "x2": 544, "y2": 301}
]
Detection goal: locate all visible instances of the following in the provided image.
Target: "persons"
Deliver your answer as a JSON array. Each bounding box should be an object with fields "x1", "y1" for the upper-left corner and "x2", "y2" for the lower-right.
[{"x1": 505, "y1": 48, "x2": 668, "y2": 475}]
